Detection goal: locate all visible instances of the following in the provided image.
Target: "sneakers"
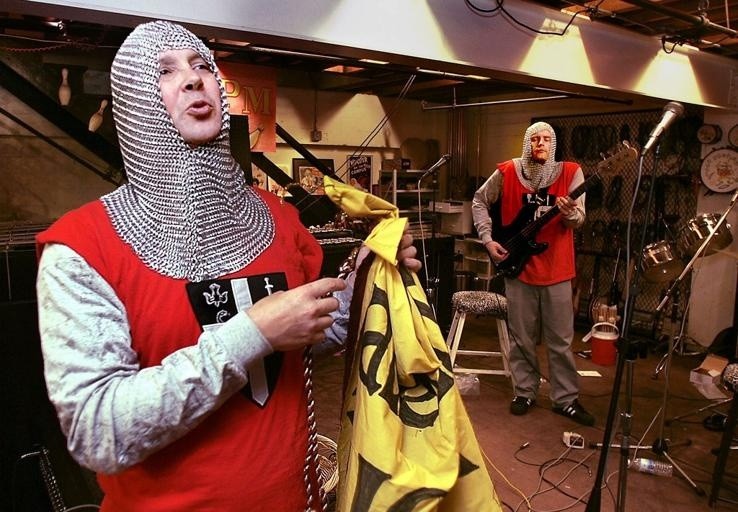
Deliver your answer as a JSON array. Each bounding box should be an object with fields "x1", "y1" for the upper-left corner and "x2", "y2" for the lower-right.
[
  {"x1": 551, "y1": 399, "x2": 595, "y2": 426},
  {"x1": 509, "y1": 393, "x2": 532, "y2": 415}
]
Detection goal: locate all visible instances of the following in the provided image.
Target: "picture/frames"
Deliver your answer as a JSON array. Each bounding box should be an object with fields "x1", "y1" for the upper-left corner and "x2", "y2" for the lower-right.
[
  {"x1": 347, "y1": 154, "x2": 374, "y2": 195},
  {"x1": 250, "y1": 157, "x2": 337, "y2": 199}
]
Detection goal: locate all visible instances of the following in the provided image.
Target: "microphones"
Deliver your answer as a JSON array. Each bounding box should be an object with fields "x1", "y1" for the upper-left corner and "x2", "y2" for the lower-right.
[
  {"x1": 640, "y1": 101, "x2": 684, "y2": 157},
  {"x1": 419, "y1": 154, "x2": 452, "y2": 182}
]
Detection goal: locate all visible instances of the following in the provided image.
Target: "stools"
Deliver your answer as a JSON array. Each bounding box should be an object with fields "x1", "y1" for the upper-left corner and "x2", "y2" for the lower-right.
[
  {"x1": 708, "y1": 362, "x2": 738, "y2": 506},
  {"x1": 445, "y1": 288, "x2": 517, "y2": 395}
]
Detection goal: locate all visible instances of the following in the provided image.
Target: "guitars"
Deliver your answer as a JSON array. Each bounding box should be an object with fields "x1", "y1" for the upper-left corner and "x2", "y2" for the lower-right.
[{"x1": 485, "y1": 140, "x2": 638, "y2": 281}]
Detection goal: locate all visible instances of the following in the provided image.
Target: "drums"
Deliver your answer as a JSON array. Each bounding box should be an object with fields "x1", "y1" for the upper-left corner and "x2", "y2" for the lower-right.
[
  {"x1": 696, "y1": 123, "x2": 722, "y2": 144},
  {"x1": 639, "y1": 239, "x2": 683, "y2": 282},
  {"x1": 728, "y1": 124, "x2": 738, "y2": 149},
  {"x1": 698, "y1": 146, "x2": 738, "y2": 193},
  {"x1": 673, "y1": 213, "x2": 733, "y2": 257}
]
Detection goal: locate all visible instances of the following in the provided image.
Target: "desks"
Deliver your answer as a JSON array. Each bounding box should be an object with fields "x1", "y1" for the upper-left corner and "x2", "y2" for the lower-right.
[{"x1": 311, "y1": 230, "x2": 454, "y2": 347}]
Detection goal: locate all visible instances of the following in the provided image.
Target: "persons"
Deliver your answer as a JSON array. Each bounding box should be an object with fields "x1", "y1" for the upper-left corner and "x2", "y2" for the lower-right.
[
  {"x1": 465, "y1": 119, "x2": 603, "y2": 432},
  {"x1": 30, "y1": 19, "x2": 427, "y2": 512}
]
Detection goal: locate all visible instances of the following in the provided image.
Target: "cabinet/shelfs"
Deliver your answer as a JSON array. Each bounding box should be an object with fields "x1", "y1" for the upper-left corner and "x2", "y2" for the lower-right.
[
  {"x1": 438, "y1": 193, "x2": 500, "y2": 293},
  {"x1": 386, "y1": 166, "x2": 437, "y2": 237}
]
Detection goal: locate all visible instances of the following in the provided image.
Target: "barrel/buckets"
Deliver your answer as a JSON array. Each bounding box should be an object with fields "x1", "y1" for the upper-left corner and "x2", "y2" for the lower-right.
[{"x1": 591, "y1": 322, "x2": 619, "y2": 367}]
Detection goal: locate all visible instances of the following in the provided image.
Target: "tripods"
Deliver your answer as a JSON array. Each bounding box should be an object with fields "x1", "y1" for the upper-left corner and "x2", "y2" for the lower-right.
[
  {"x1": 588, "y1": 203, "x2": 733, "y2": 496},
  {"x1": 666, "y1": 385, "x2": 738, "y2": 512}
]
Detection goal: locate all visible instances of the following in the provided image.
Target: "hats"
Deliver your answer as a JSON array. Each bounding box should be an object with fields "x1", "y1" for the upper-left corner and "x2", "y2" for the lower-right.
[
  {"x1": 512, "y1": 121, "x2": 564, "y2": 192},
  {"x1": 97, "y1": 20, "x2": 277, "y2": 283}
]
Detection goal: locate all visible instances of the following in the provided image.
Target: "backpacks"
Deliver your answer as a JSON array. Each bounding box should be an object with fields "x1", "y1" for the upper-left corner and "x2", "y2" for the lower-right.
[{"x1": 302, "y1": 175, "x2": 507, "y2": 512}]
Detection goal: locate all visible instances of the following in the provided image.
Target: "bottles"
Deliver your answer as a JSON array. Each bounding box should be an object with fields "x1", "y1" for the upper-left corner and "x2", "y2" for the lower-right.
[{"x1": 625, "y1": 458, "x2": 674, "y2": 478}]
